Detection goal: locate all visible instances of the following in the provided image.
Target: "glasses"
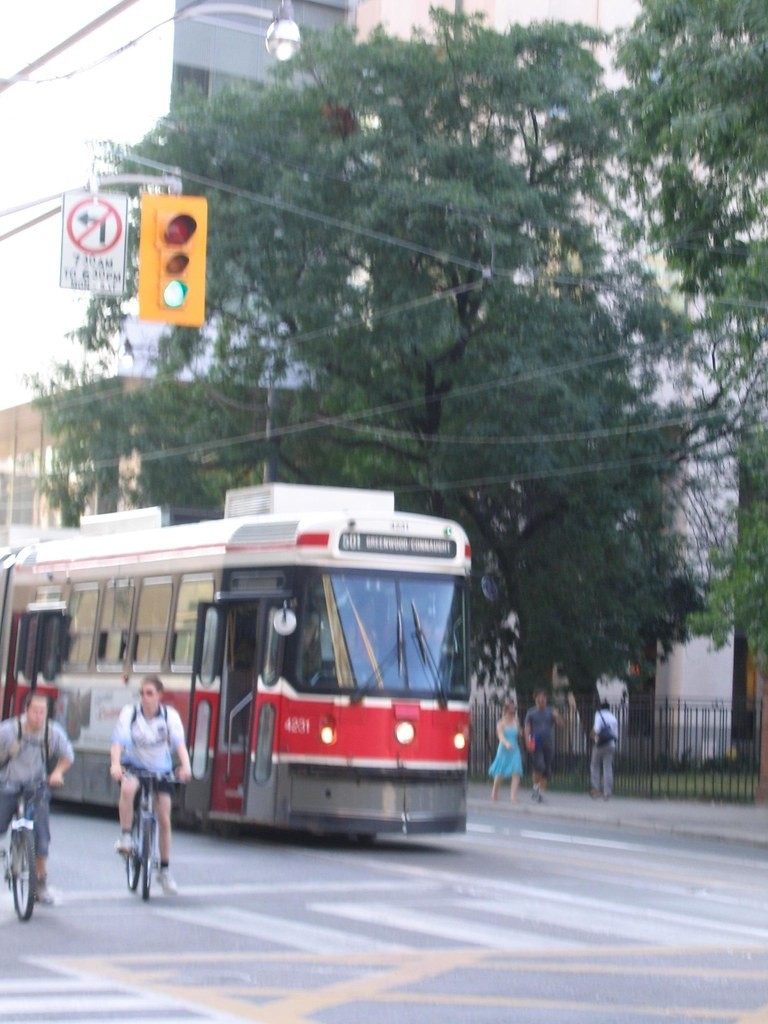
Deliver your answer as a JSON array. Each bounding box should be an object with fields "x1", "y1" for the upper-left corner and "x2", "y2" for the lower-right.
[{"x1": 140, "y1": 690, "x2": 157, "y2": 696}]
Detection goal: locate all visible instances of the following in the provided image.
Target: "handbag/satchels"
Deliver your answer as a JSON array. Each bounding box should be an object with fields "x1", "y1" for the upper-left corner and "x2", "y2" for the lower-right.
[
  {"x1": 595, "y1": 726, "x2": 613, "y2": 746},
  {"x1": 530, "y1": 736, "x2": 536, "y2": 752}
]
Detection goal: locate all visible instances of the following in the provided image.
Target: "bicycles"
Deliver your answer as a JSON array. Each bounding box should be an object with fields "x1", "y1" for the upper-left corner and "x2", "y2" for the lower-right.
[
  {"x1": 6, "y1": 781, "x2": 51, "y2": 917},
  {"x1": 123, "y1": 763, "x2": 181, "y2": 901}
]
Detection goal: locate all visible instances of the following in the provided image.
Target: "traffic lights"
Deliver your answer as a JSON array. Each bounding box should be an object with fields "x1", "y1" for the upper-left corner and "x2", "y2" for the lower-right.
[{"x1": 139, "y1": 197, "x2": 208, "y2": 325}]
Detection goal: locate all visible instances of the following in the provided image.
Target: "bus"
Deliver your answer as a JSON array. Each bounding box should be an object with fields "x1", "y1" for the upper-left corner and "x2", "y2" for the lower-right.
[{"x1": 0, "y1": 484, "x2": 504, "y2": 849}]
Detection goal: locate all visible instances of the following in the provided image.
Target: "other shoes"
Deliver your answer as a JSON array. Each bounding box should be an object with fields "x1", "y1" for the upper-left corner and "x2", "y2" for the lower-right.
[
  {"x1": 531, "y1": 784, "x2": 544, "y2": 804},
  {"x1": 157, "y1": 873, "x2": 179, "y2": 897},
  {"x1": 35, "y1": 881, "x2": 54, "y2": 903},
  {"x1": 603, "y1": 792, "x2": 609, "y2": 801},
  {"x1": 117, "y1": 832, "x2": 132, "y2": 853},
  {"x1": 591, "y1": 790, "x2": 601, "y2": 799}
]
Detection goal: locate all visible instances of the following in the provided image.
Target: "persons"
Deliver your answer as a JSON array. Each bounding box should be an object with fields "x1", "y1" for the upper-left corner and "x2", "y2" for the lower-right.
[
  {"x1": 110, "y1": 675, "x2": 192, "y2": 896},
  {"x1": 491, "y1": 703, "x2": 524, "y2": 805},
  {"x1": 524, "y1": 687, "x2": 565, "y2": 803},
  {"x1": 589, "y1": 703, "x2": 618, "y2": 802},
  {"x1": 0, "y1": 692, "x2": 74, "y2": 904}
]
